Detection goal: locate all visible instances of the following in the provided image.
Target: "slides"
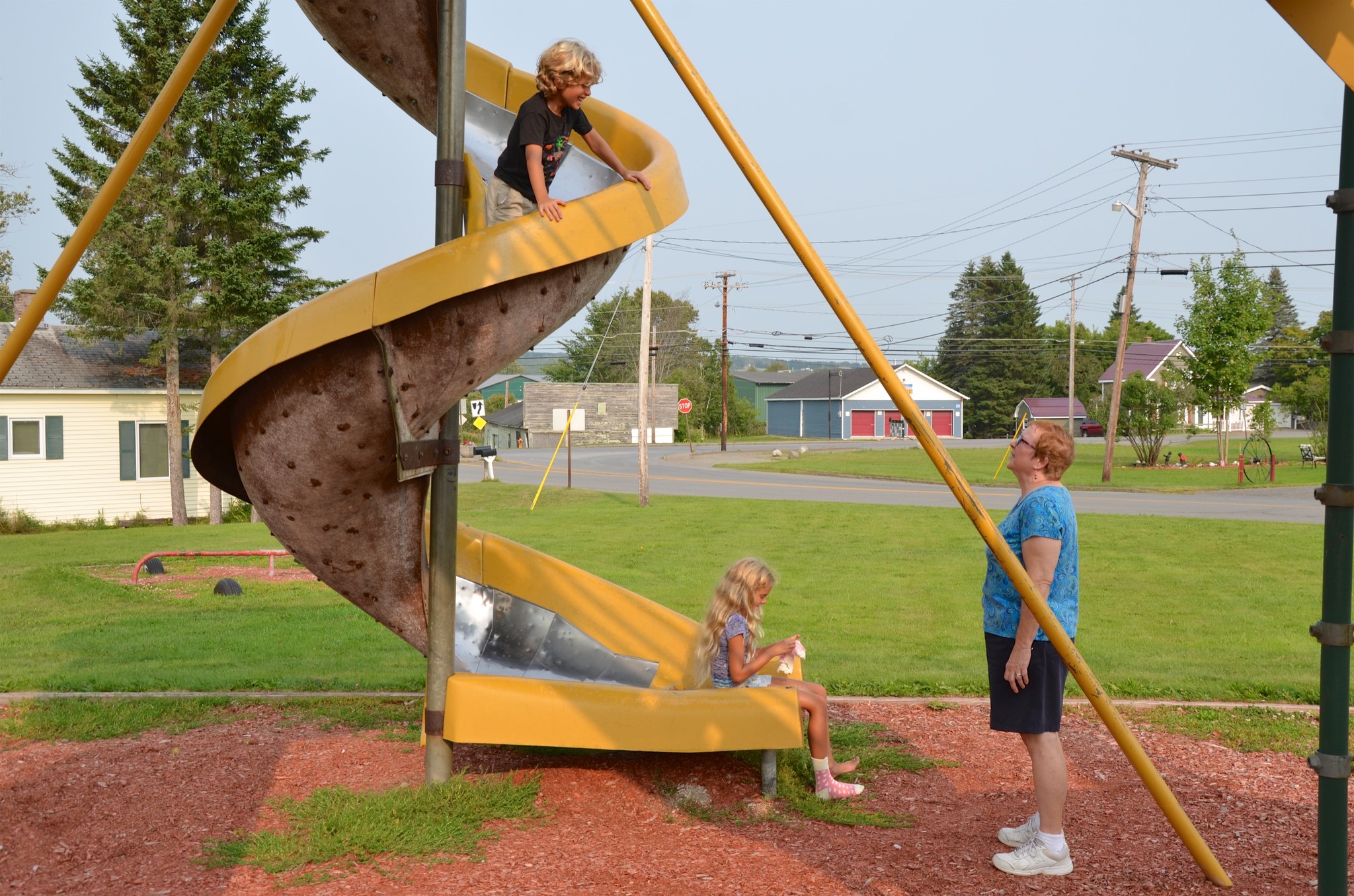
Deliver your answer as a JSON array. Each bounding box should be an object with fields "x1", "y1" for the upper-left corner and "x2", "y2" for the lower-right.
[{"x1": 190, "y1": 1, "x2": 802, "y2": 751}]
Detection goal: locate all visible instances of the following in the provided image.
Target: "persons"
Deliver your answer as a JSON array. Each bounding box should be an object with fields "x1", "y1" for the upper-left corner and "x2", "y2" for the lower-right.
[
  {"x1": 484, "y1": 39, "x2": 652, "y2": 222},
  {"x1": 979, "y1": 421, "x2": 1081, "y2": 877},
  {"x1": 693, "y1": 557, "x2": 864, "y2": 800}
]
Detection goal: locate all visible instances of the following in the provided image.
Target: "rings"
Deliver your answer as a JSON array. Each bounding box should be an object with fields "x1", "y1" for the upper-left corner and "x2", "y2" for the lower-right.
[{"x1": 1015, "y1": 673, "x2": 1022, "y2": 679}]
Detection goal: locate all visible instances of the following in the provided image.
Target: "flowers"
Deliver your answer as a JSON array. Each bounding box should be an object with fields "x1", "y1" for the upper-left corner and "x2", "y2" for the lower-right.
[{"x1": 460, "y1": 440, "x2": 475, "y2": 446}]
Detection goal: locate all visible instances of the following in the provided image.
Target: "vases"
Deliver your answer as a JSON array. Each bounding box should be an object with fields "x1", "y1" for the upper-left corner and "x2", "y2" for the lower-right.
[{"x1": 459, "y1": 445, "x2": 478, "y2": 458}]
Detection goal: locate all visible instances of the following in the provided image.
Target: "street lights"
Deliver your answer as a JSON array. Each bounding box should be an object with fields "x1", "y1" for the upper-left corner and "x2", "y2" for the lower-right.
[{"x1": 713, "y1": 302, "x2": 728, "y2": 452}]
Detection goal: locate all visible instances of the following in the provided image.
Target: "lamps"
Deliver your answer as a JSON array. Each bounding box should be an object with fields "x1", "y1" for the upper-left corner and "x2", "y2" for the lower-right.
[{"x1": 902, "y1": 378, "x2": 908, "y2": 385}]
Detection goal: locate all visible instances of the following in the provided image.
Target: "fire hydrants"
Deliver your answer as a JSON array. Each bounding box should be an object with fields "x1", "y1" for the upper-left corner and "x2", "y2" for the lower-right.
[{"x1": 517, "y1": 438, "x2": 524, "y2": 448}]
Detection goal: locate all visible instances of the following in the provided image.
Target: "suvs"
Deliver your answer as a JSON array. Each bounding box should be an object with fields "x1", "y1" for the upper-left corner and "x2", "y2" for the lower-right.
[{"x1": 1079, "y1": 417, "x2": 1133, "y2": 437}]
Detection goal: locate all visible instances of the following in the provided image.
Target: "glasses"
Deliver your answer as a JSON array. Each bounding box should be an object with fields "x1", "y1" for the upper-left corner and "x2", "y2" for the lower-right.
[{"x1": 1016, "y1": 434, "x2": 1037, "y2": 451}]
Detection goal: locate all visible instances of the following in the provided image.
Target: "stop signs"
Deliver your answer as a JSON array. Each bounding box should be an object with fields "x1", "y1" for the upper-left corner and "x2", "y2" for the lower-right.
[{"x1": 677, "y1": 398, "x2": 692, "y2": 414}]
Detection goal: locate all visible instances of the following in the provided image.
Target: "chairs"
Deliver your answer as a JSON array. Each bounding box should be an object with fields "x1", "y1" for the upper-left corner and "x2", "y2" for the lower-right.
[{"x1": 1298, "y1": 444, "x2": 1326, "y2": 470}]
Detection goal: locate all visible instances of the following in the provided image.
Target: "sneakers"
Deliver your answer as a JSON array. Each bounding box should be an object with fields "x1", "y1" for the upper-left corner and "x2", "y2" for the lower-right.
[
  {"x1": 992, "y1": 831, "x2": 1073, "y2": 875},
  {"x1": 997, "y1": 813, "x2": 1035, "y2": 847}
]
